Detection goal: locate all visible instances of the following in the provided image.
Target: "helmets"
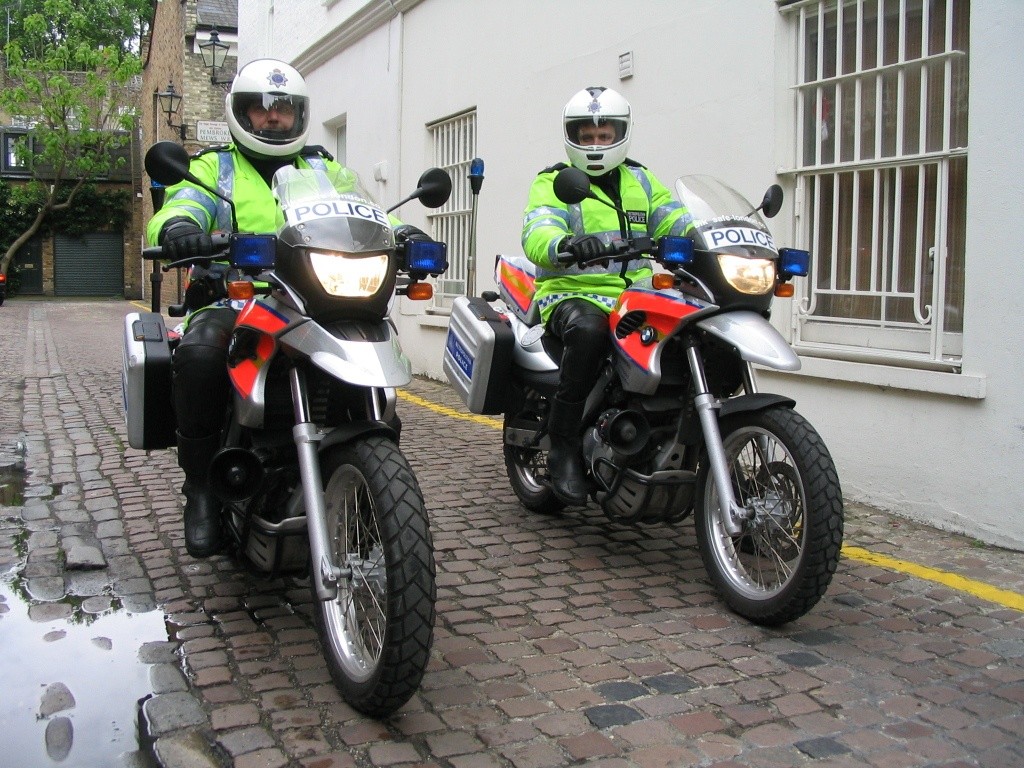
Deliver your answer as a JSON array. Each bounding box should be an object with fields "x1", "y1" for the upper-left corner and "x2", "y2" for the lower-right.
[
  {"x1": 225, "y1": 57, "x2": 311, "y2": 159},
  {"x1": 562, "y1": 85, "x2": 632, "y2": 176}
]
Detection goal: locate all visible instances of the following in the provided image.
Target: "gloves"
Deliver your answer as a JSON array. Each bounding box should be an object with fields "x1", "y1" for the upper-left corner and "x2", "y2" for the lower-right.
[
  {"x1": 562, "y1": 234, "x2": 609, "y2": 269},
  {"x1": 398, "y1": 226, "x2": 442, "y2": 280},
  {"x1": 166, "y1": 222, "x2": 212, "y2": 269}
]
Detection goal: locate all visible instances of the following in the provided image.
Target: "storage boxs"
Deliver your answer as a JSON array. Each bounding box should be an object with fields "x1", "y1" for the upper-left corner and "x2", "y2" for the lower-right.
[
  {"x1": 496, "y1": 253, "x2": 540, "y2": 324},
  {"x1": 443, "y1": 292, "x2": 528, "y2": 414},
  {"x1": 121, "y1": 313, "x2": 171, "y2": 449}
]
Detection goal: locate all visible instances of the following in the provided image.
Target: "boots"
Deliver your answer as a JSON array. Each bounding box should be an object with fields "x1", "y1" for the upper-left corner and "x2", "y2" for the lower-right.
[
  {"x1": 543, "y1": 394, "x2": 588, "y2": 505},
  {"x1": 174, "y1": 427, "x2": 221, "y2": 559}
]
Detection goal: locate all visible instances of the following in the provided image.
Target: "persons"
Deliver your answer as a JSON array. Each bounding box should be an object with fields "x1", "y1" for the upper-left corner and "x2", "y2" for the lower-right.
[
  {"x1": 520, "y1": 85, "x2": 793, "y2": 507},
  {"x1": 142, "y1": 59, "x2": 438, "y2": 558}
]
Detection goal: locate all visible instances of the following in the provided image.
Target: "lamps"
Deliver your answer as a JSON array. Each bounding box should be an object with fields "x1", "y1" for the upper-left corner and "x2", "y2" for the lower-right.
[
  {"x1": 158, "y1": 80, "x2": 198, "y2": 140},
  {"x1": 200, "y1": 22, "x2": 238, "y2": 96}
]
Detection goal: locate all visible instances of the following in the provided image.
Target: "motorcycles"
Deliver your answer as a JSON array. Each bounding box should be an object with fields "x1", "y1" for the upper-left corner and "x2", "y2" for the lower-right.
[
  {"x1": 443, "y1": 167, "x2": 845, "y2": 630},
  {"x1": 120, "y1": 140, "x2": 452, "y2": 723}
]
